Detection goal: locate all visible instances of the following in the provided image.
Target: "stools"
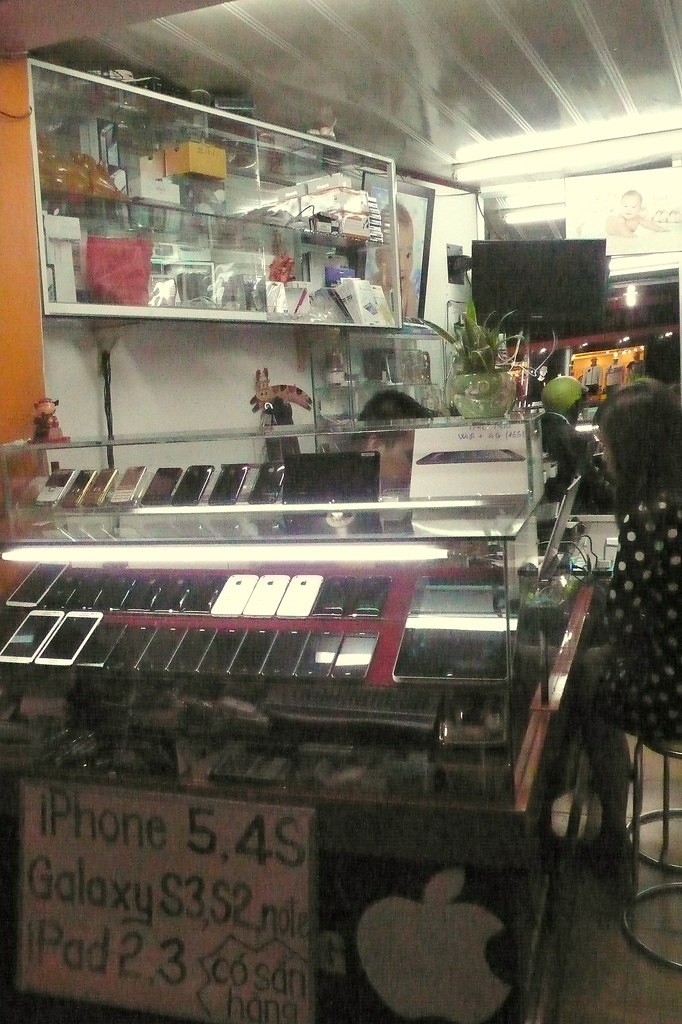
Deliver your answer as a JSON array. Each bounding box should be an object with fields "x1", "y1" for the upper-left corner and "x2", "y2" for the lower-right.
[{"x1": 623, "y1": 738, "x2": 682, "y2": 969}]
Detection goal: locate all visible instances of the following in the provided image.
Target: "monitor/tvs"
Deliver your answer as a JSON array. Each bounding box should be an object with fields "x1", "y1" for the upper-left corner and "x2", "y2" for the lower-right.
[{"x1": 471, "y1": 239, "x2": 606, "y2": 322}]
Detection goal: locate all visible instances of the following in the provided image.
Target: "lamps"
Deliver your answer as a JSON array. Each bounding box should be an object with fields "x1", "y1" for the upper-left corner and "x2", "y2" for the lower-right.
[{"x1": 622, "y1": 285, "x2": 638, "y2": 307}]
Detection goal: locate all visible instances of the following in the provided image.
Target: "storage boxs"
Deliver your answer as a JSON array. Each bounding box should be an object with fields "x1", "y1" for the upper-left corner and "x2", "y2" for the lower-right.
[{"x1": 164, "y1": 141, "x2": 228, "y2": 181}]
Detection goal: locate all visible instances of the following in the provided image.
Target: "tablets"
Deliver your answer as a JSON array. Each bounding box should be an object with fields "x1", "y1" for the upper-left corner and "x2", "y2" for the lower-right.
[
  {"x1": 392, "y1": 575, "x2": 522, "y2": 685},
  {"x1": 416, "y1": 449, "x2": 526, "y2": 464}
]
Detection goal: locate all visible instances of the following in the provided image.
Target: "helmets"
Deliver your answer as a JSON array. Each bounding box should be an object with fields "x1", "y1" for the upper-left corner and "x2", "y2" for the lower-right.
[{"x1": 541, "y1": 376, "x2": 589, "y2": 415}]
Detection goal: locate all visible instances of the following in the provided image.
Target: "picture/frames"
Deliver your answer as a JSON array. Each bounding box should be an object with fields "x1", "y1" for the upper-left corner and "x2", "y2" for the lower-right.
[{"x1": 352, "y1": 172, "x2": 435, "y2": 332}]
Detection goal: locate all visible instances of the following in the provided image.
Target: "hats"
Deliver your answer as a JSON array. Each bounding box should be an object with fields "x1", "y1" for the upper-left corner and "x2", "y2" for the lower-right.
[{"x1": 612, "y1": 353, "x2": 620, "y2": 360}]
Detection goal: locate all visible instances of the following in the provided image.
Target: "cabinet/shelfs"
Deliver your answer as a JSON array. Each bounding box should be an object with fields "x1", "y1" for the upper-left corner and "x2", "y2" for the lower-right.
[
  {"x1": 27, "y1": 60, "x2": 402, "y2": 330},
  {"x1": 2, "y1": 417, "x2": 594, "y2": 1024}
]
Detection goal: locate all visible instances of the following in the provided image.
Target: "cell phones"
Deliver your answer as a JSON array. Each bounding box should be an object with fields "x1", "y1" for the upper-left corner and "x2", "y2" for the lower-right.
[{"x1": 0, "y1": 450, "x2": 386, "y2": 683}]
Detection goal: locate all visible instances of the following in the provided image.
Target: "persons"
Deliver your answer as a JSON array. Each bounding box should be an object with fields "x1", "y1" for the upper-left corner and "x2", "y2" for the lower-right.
[
  {"x1": 569, "y1": 377, "x2": 682, "y2": 876},
  {"x1": 343, "y1": 390, "x2": 439, "y2": 490},
  {"x1": 603, "y1": 188, "x2": 672, "y2": 238},
  {"x1": 368, "y1": 201, "x2": 418, "y2": 322},
  {"x1": 576, "y1": 342, "x2": 647, "y2": 400}
]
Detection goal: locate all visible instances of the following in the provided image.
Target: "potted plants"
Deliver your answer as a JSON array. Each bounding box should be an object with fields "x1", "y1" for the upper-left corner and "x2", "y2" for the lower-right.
[{"x1": 404, "y1": 298, "x2": 520, "y2": 422}]
